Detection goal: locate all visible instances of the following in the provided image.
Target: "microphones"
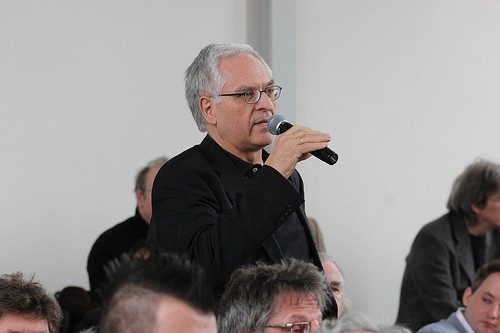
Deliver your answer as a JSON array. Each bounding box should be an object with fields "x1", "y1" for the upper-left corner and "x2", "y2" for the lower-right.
[{"x1": 268, "y1": 115, "x2": 338, "y2": 165}]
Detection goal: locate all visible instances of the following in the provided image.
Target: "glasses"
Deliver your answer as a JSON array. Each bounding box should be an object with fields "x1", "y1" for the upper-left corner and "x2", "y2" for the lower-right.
[
  {"x1": 209, "y1": 86, "x2": 282, "y2": 103},
  {"x1": 263, "y1": 322, "x2": 322, "y2": 333}
]
